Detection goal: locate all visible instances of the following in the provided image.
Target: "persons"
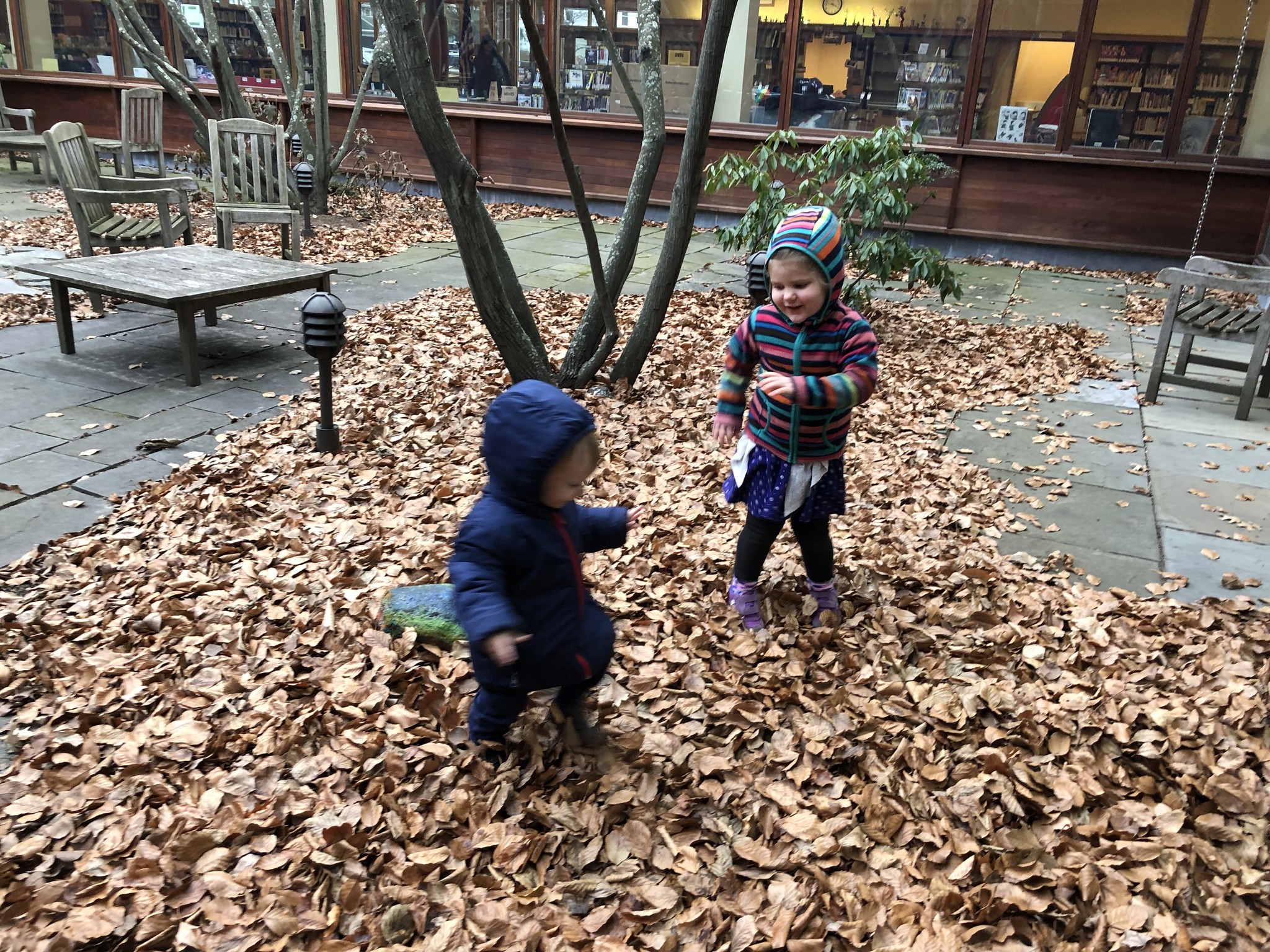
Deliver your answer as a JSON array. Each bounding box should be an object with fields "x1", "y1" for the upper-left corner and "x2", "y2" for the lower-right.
[
  {"x1": 713, "y1": 206, "x2": 880, "y2": 634},
  {"x1": 448, "y1": 378, "x2": 646, "y2": 765},
  {"x1": 471, "y1": 35, "x2": 512, "y2": 99},
  {"x1": 55, "y1": 33, "x2": 92, "y2": 72},
  {"x1": 1031, "y1": 73, "x2": 1086, "y2": 135}
]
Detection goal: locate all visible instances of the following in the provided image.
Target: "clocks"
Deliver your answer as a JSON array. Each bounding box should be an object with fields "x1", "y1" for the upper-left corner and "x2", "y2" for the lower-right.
[{"x1": 822, "y1": 0, "x2": 843, "y2": 15}]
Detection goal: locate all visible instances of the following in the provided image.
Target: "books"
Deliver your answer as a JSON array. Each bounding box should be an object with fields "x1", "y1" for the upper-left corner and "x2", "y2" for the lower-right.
[
  {"x1": 516, "y1": 46, "x2": 640, "y2": 113},
  {"x1": 1084, "y1": 64, "x2": 1247, "y2": 156},
  {"x1": 48, "y1": 0, "x2": 314, "y2": 85},
  {"x1": 896, "y1": 60, "x2": 987, "y2": 135}
]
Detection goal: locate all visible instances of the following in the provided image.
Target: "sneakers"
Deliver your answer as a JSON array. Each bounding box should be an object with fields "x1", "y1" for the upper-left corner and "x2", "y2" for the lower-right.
[
  {"x1": 729, "y1": 573, "x2": 765, "y2": 634},
  {"x1": 806, "y1": 578, "x2": 841, "y2": 630}
]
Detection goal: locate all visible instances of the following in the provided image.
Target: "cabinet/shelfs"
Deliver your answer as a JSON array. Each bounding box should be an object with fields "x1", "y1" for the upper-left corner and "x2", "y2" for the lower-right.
[
  {"x1": 49, "y1": 0, "x2": 313, "y2": 76},
  {"x1": 867, "y1": 35, "x2": 993, "y2": 114},
  {"x1": 521, "y1": 0, "x2": 641, "y2": 95},
  {"x1": 1085, "y1": 42, "x2": 1263, "y2": 142}
]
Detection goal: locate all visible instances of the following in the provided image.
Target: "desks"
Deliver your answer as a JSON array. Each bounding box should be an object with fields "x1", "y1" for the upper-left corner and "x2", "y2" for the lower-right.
[
  {"x1": 0, "y1": 135, "x2": 53, "y2": 186},
  {"x1": 15, "y1": 243, "x2": 338, "y2": 387}
]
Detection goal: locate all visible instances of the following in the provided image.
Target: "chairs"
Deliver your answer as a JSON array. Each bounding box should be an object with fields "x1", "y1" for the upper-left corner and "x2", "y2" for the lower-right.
[
  {"x1": 94, "y1": 87, "x2": 167, "y2": 191},
  {"x1": 207, "y1": 119, "x2": 300, "y2": 262},
  {"x1": 260, "y1": 68, "x2": 276, "y2": 79},
  {"x1": 0, "y1": 84, "x2": 41, "y2": 174},
  {"x1": 1145, "y1": 256, "x2": 1270, "y2": 420},
  {"x1": 43, "y1": 122, "x2": 200, "y2": 314}
]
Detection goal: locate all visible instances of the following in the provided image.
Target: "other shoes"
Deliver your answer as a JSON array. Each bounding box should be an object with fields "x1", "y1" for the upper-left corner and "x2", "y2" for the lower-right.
[
  {"x1": 552, "y1": 705, "x2": 594, "y2": 747},
  {"x1": 477, "y1": 740, "x2": 508, "y2": 773}
]
